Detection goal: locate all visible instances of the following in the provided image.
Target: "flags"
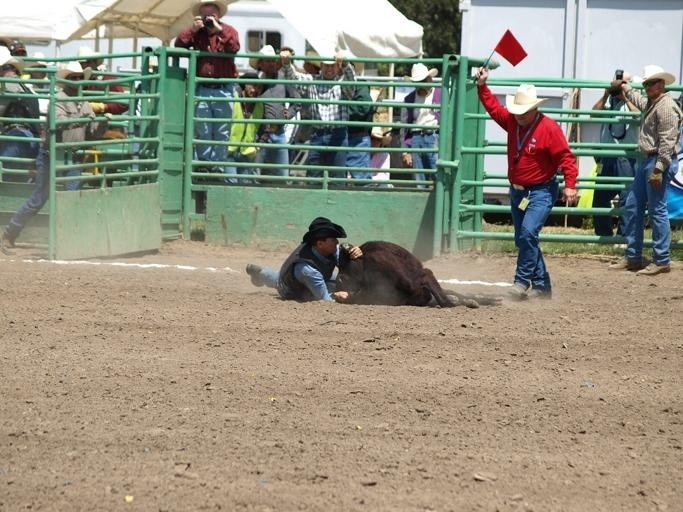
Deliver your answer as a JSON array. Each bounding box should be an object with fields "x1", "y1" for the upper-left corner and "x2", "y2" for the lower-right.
[{"x1": 494, "y1": 28, "x2": 527, "y2": 67}]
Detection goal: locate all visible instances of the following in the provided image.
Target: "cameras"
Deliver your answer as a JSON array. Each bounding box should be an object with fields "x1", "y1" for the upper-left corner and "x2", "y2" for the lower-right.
[
  {"x1": 202, "y1": 17, "x2": 214, "y2": 28},
  {"x1": 609, "y1": 70, "x2": 624, "y2": 97}
]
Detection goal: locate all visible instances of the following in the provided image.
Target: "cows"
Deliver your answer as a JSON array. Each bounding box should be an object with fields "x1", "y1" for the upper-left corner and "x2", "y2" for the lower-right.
[{"x1": 336, "y1": 241, "x2": 502, "y2": 307}]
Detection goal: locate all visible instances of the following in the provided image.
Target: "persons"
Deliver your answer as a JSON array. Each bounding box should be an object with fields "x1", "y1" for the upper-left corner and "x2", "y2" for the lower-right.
[
  {"x1": 475, "y1": 66, "x2": 578, "y2": 301},
  {"x1": 246, "y1": 217, "x2": 363, "y2": 303},
  {"x1": 588, "y1": 72, "x2": 640, "y2": 249},
  {"x1": 0, "y1": 61, "x2": 113, "y2": 256},
  {"x1": 607, "y1": 66, "x2": 682, "y2": 276}
]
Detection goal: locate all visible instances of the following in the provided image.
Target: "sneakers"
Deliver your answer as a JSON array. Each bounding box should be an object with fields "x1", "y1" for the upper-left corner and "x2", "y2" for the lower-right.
[
  {"x1": 635, "y1": 262, "x2": 671, "y2": 276},
  {"x1": 523, "y1": 288, "x2": 551, "y2": 300},
  {"x1": 0, "y1": 231, "x2": 17, "y2": 255},
  {"x1": 608, "y1": 259, "x2": 642, "y2": 271},
  {"x1": 508, "y1": 283, "x2": 528, "y2": 298},
  {"x1": 246, "y1": 264, "x2": 264, "y2": 287}
]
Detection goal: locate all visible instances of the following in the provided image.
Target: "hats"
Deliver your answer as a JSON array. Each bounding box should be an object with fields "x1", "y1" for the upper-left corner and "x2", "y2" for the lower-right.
[
  {"x1": 75, "y1": 47, "x2": 104, "y2": 66},
  {"x1": 250, "y1": 45, "x2": 283, "y2": 70},
  {"x1": 192, "y1": 0, "x2": 227, "y2": 19},
  {"x1": 404, "y1": 63, "x2": 439, "y2": 82},
  {"x1": 25, "y1": 52, "x2": 53, "y2": 67},
  {"x1": 506, "y1": 83, "x2": 550, "y2": 116},
  {"x1": 56, "y1": 61, "x2": 92, "y2": 88},
  {"x1": 0, "y1": 46, "x2": 23, "y2": 73},
  {"x1": 633, "y1": 65, "x2": 676, "y2": 85},
  {"x1": 302, "y1": 217, "x2": 347, "y2": 242}
]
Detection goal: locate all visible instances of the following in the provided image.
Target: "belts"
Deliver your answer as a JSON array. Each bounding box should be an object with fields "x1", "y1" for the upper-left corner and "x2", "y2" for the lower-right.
[
  {"x1": 413, "y1": 129, "x2": 439, "y2": 135},
  {"x1": 511, "y1": 176, "x2": 556, "y2": 191}
]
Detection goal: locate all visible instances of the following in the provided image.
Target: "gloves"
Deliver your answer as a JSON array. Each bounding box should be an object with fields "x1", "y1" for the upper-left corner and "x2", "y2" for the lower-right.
[{"x1": 647, "y1": 170, "x2": 663, "y2": 192}]
